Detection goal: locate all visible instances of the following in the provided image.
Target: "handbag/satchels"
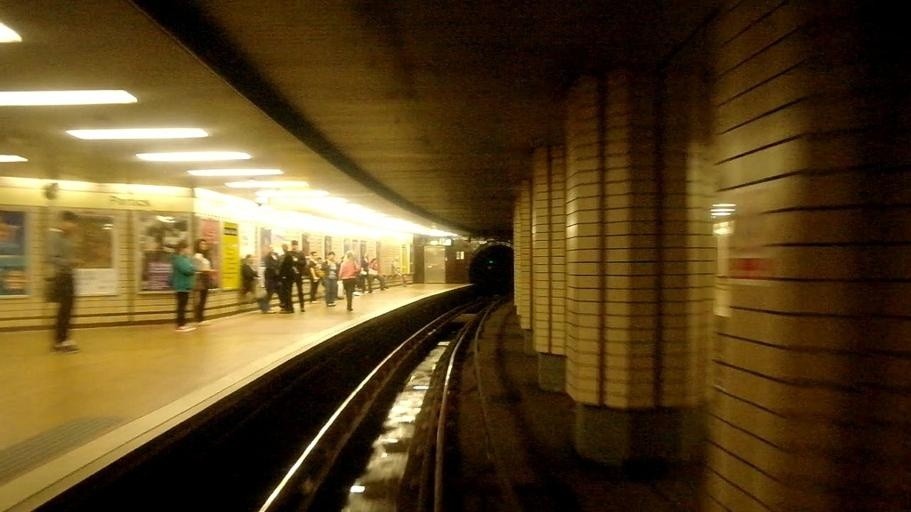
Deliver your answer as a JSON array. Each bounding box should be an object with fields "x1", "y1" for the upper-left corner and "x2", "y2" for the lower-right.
[{"x1": 255, "y1": 285, "x2": 267, "y2": 299}]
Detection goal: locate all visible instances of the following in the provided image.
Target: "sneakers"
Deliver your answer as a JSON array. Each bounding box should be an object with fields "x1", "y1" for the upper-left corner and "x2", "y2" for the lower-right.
[
  {"x1": 52, "y1": 340, "x2": 81, "y2": 353},
  {"x1": 262, "y1": 308, "x2": 305, "y2": 313},
  {"x1": 326, "y1": 300, "x2": 336, "y2": 306},
  {"x1": 176, "y1": 320, "x2": 210, "y2": 332}
]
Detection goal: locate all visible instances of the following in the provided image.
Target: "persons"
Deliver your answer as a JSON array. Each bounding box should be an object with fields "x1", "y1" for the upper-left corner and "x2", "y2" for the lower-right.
[
  {"x1": 193, "y1": 238, "x2": 219, "y2": 323},
  {"x1": 43, "y1": 209, "x2": 86, "y2": 354},
  {"x1": 240, "y1": 237, "x2": 388, "y2": 312},
  {"x1": 169, "y1": 240, "x2": 209, "y2": 332}
]
